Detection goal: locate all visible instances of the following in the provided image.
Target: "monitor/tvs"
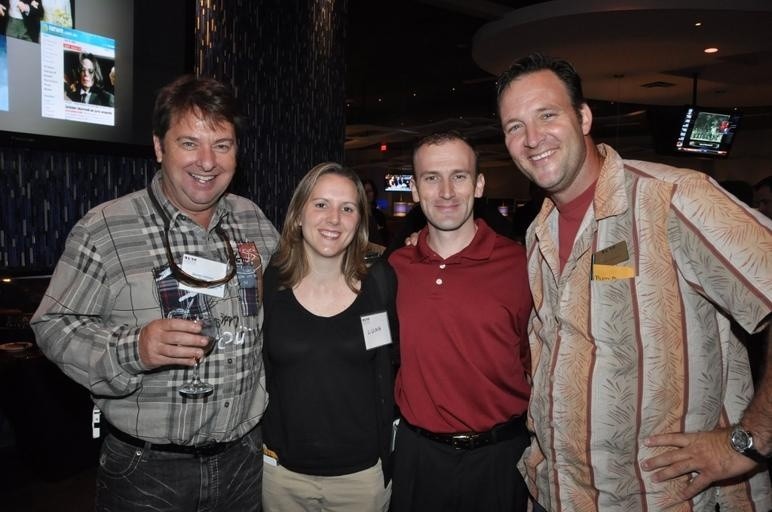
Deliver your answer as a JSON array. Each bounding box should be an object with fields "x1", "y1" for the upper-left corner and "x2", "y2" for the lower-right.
[
  {"x1": 383, "y1": 169, "x2": 416, "y2": 194},
  {"x1": 673, "y1": 102, "x2": 742, "y2": 160},
  {"x1": 0, "y1": 0, "x2": 149, "y2": 160}
]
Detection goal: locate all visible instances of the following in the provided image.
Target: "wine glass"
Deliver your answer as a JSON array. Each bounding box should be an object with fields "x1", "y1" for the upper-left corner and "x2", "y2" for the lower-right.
[{"x1": 178, "y1": 317, "x2": 217, "y2": 395}]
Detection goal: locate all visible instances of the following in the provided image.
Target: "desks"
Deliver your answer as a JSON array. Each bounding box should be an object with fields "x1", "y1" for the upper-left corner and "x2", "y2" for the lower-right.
[{"x1": 0, "y1": 336, "x2": 95, "y2": 473}]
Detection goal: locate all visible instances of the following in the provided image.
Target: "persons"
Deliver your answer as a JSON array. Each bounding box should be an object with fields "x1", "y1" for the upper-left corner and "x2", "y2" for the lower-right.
[
  {"x1": 362, "y1": 178, "x2": 387, "y2": 236},
  {"x1": 495, "y1": 52, "x2": 772, "y2": 512},
  {"x1": 0, "y1": 0, "x2": 44, "y2": 43},
  {"x1": 257, "y1": 162, "x2": 401, "y2": 512},
  {"x1": 64, "y1": 53, "x2": 115, "y2": 107},
  {"x1": 30, "y1": 75, "x2": 283, "y2": 512},
  {"x1": 387, "y1": 132, "x2": 534, "y2": 512}
]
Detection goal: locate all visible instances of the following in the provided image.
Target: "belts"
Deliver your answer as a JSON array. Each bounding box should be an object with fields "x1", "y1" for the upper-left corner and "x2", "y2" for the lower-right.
[
  {"x1": 109, "y1": 423, "x2": 241, "y2": 456},
  {"x1": 407, "y1": 422, "x2": 495, "y2": 453}
]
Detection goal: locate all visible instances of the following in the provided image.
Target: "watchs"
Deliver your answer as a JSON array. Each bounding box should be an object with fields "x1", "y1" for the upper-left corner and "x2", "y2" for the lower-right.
[{"x1": 730, "y1": 422, "x2": 772, "y2": 468}]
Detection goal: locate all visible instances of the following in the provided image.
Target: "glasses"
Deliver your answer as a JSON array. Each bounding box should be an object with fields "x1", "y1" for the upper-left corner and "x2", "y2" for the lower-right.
[{"x1": 165, "y1": 229, "x2": 236, "y2": 290}]
